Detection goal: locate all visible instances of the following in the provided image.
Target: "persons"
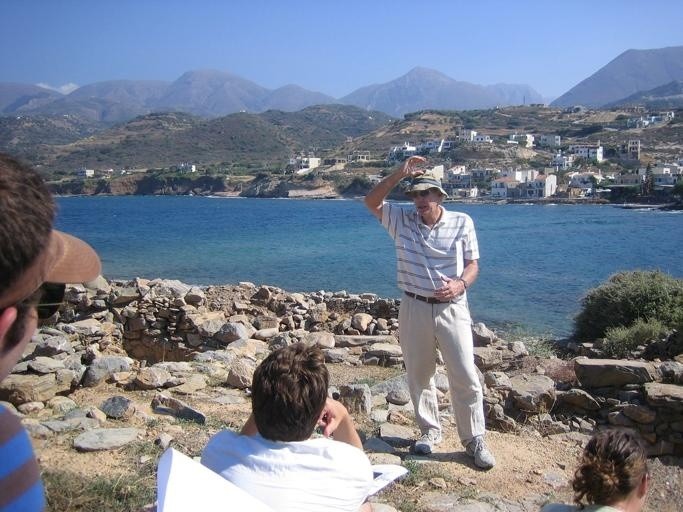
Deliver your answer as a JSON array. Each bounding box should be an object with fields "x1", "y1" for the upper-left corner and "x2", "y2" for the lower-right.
[
  {"x1": 535, "y1": 425, "x2": 650, "y2": 512},
  {"x1": 198, "y1": 340, "x2": 372, "y2": 512},
  {"x1": 362, "y1": 155, "x2": 497, "y2": 469},
  {"x1": 0, "y1": 151, "x2": 101, "y2": 512}
]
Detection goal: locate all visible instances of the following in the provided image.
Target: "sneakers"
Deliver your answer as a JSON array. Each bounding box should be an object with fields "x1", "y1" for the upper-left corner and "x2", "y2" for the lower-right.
[
  {"x1": 465, "y1": 435, "x2": 496, "y2": 468},
  {"x1": 414, "y1": 433, "x2": 442, "y2": 454}
]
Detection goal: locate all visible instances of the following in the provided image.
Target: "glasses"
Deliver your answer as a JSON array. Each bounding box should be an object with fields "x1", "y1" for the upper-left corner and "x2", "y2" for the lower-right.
[
  {"x1": 16, "y1": 283, "x2": 63, "y2": 319},
  {"x1": 410, "y1": 189, "x2": 436, "y2": 197}
]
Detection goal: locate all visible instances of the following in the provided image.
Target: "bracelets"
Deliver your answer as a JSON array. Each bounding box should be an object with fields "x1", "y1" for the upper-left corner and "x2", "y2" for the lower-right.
[{"x1": 458, "y1": 278, "x2": 469, "y2": 290}]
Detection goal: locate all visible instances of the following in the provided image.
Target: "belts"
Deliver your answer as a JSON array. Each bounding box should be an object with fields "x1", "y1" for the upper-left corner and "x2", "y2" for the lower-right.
[{"x1": 404, "y1": 291, "x2": 464, "y2": 303}]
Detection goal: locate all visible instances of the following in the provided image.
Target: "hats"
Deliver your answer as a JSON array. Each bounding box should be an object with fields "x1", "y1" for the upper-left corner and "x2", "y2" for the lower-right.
[
  {"x1": 0, "y1": 230, "x2": 101, "y2": 310},
  {"x1": 404, "y1": 170, "x2": 449, "y2": 200}
]
